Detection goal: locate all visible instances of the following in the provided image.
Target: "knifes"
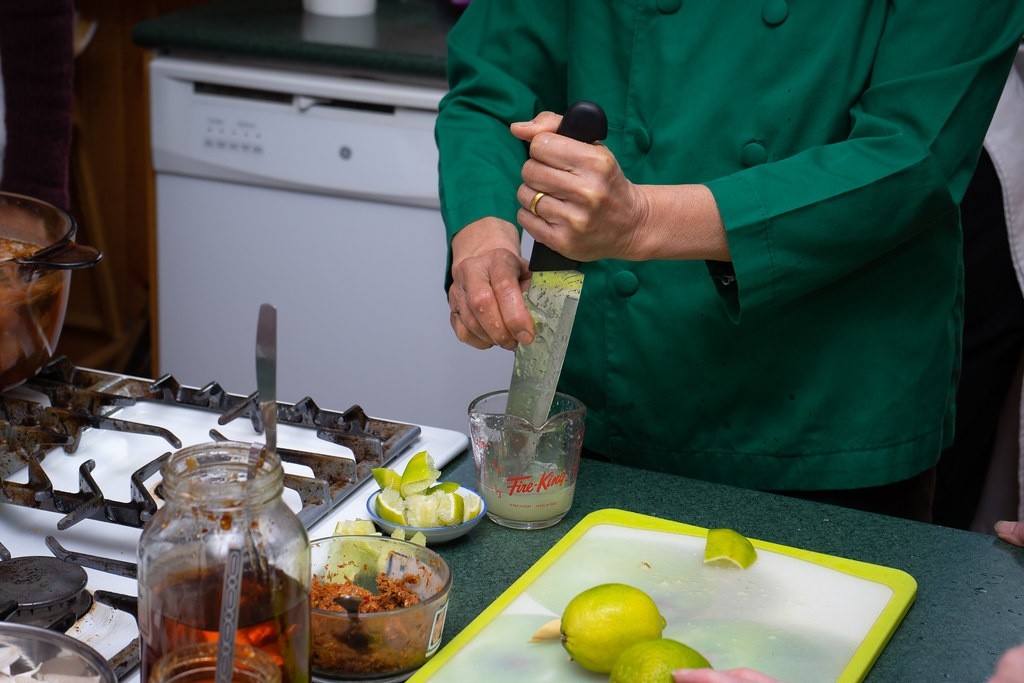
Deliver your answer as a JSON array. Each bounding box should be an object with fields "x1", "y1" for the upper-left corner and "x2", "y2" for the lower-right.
[{"x1": 501, "y1": 96, "x2": 608, "y2": 479}]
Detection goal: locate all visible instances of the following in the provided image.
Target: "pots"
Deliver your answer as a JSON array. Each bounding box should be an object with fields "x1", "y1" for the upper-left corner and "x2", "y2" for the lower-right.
[{"x1": 1, "y1": 187, "x2": 104, "y2": 392}]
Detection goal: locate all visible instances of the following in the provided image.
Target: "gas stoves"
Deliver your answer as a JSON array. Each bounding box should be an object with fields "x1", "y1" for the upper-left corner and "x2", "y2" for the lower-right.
[{"x1": 2, "y1": 368, "x2": 470, "y2": 680}]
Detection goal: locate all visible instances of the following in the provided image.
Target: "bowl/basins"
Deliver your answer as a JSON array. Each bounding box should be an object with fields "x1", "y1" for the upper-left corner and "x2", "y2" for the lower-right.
[
  {"x1": 0, "y1": 623, "x2": 116, "y2": 683},
  {"x1": 308, "y1": 530, "x2": 453, "y2": 683},
  {"x1": 364, "y1": 481, "x2": 489, "y2": 546}
]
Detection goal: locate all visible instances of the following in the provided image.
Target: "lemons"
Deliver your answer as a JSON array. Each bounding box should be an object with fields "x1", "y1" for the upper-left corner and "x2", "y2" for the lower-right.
[
  {"x1": 559, "y1": 582, "x2": 717, "y2": 683},
  {"x1": 370, "y1": 450, "x2": 481, "y2": 527}
]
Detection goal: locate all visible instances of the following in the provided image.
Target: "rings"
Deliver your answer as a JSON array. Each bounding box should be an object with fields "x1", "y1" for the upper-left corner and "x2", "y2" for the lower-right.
[{"x1": 530, "y1": 193, "x2": 547, "y2": 217}]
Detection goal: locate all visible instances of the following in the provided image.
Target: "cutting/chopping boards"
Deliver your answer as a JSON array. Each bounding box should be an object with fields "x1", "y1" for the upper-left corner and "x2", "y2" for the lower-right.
[{"x1": 397, "y1": 507, "x2": 919, "y2": 683}]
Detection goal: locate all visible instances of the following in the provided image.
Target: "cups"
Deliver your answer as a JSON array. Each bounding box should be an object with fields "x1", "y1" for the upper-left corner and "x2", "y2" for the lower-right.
[{"x1": 469, "y1": 390, "x2": 586, "y2": 532}]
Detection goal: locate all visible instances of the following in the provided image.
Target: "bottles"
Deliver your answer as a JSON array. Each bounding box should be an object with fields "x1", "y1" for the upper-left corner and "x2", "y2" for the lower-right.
[{"x1": 136, "y1": 445, "x2": 312, "y2": 681}]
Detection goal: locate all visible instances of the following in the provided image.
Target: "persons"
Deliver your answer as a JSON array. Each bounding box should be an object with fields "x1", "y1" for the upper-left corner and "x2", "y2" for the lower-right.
[
  {"x1": 435, "y1": 0, "x2": 1024, "y2": 531},
  {"x1": 989, "y1": 517, "x2": 1024, "y2": 683}
]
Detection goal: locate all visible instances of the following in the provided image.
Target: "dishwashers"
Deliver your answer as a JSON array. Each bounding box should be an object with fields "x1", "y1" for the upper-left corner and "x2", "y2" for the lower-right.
[{"x1": 144, "y1": 55, "x2": 536, "y2": 436}]
